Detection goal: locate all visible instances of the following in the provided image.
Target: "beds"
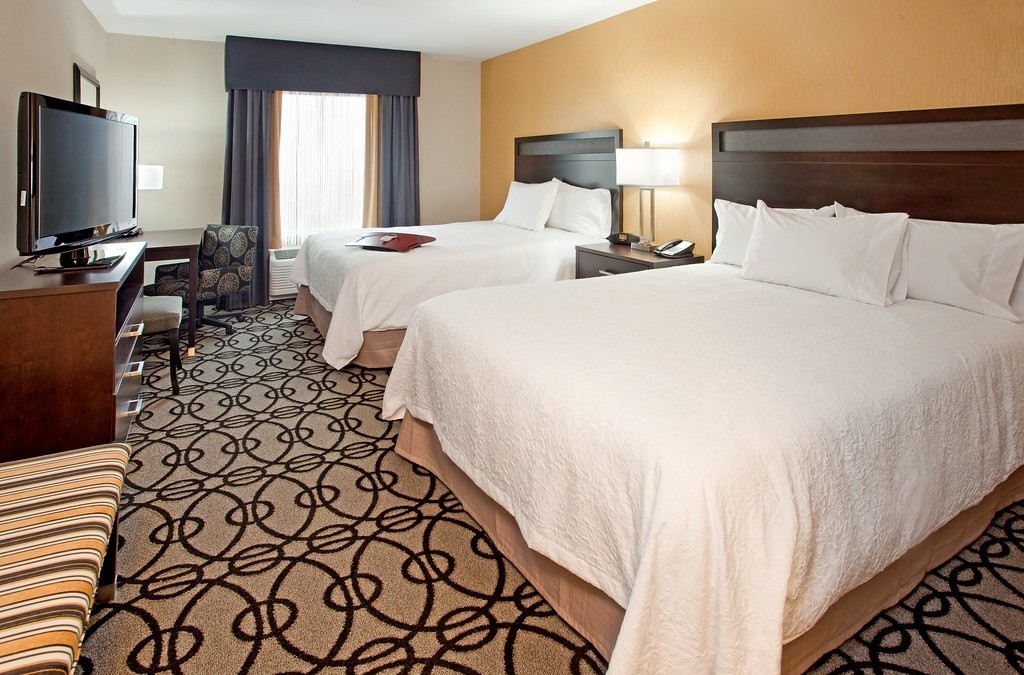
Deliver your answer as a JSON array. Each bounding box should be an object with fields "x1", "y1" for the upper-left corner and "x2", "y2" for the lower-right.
[
  {"x1": 290, "y1": 127, "x2": 626, "y2": 371},
  {"x1": 382, "y1": 103, "x2": 1024, "y2": 675}
]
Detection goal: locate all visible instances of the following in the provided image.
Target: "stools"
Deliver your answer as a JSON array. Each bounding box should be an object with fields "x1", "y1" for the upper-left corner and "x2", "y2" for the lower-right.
[{"x1": 143, "y1": 297, "x2": 184, "y2": 397}]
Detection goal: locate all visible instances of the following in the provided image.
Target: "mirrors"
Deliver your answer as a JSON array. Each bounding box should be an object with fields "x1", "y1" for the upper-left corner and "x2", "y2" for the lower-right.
[{"x1": 72, "y1": 62, "x2": 102, "y2": 108}]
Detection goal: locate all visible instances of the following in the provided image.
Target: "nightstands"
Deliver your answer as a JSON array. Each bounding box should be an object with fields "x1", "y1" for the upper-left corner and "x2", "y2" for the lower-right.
[{"x1": 575, "y1": 239, "x2": 704, "y2": 279}]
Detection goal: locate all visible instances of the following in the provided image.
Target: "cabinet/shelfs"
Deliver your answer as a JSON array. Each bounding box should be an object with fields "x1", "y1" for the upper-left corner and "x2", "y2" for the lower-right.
[{"x1": 0, "y1": 241, "x2": 148, "y2": 464}]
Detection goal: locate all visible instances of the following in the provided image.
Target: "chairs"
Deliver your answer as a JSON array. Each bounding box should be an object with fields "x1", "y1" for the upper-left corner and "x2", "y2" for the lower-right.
[{"x1": 142, "y1": 224, "x2": 259, "y2": 336}]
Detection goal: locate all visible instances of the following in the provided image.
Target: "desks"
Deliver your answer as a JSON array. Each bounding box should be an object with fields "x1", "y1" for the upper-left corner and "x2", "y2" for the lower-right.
[{"x1": 143, "y1": 228, "x2": 205, "y2": 356}]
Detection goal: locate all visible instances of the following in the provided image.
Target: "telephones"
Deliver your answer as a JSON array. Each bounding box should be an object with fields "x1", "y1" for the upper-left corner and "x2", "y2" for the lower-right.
[{"x1": 653, "y1": 239, "x2": 695, "y2": 258}]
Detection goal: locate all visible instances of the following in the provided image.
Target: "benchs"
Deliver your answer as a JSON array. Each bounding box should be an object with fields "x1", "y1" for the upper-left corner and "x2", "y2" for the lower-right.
[{"x1": 0, "y1": 443, "x2": 132, "y2": 674}]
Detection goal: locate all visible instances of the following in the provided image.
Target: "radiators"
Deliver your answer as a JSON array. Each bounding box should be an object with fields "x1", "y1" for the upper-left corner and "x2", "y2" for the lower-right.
[{"x1": 269, "y1": 245, "x2": 302, "y2": 302}]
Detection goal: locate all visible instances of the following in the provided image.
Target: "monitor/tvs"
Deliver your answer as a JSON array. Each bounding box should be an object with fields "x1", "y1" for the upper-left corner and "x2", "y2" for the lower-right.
[{"x1": 17, "y1": 89, "x2": 138, "y2": 272}]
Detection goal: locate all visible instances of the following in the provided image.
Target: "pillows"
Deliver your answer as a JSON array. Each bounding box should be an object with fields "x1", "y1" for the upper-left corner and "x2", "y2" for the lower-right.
[
  {"x1": 546, "y1": 177, "x2": 612, "y2": 238},
  {"x1": 740, "y1": 199, "x2": 909, "y2": 308},
  {"x1": 831, "y1": 201, "x2": 1024, "y2": 323},
  {"x1": 710, "y1": 198, "x2": 834, "y2": 268},
  {"x1": 493, "y1": 179, "x2": 561, "y2": 231}
]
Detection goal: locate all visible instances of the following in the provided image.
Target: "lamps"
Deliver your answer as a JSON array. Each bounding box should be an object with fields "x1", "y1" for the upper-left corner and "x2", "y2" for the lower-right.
[
  {"x1": 139, "y1": 164, "x2": 164, "y2": 190},
  {"x1": 614, "y1": 141, "x2": 681, "y2": 253}
]
